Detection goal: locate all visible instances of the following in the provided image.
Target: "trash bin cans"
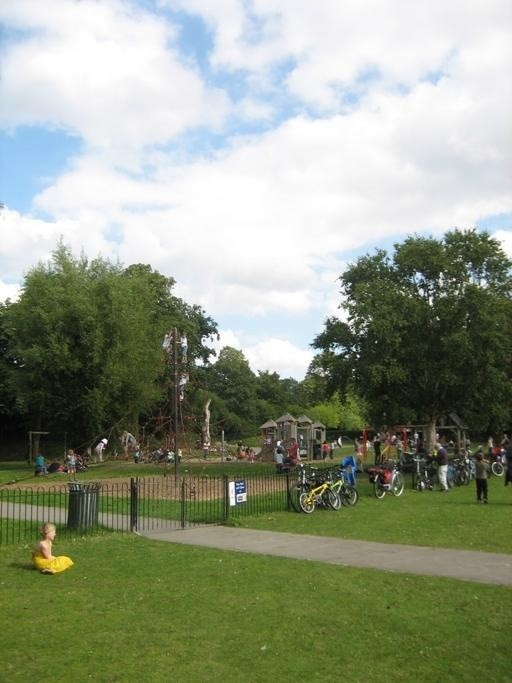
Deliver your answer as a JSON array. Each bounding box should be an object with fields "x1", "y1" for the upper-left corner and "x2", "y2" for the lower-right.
[
  {"x1": 313, "y1": 443, "x2": 322, "y2": 460},
  {"x1": 68, "y1": 481, "x2": 99, "y2": 529}
]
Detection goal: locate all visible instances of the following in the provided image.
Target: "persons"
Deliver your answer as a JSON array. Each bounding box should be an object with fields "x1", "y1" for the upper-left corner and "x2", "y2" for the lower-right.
[
  {"x1": 33, "y1": 523, "x2": 74, "y2": 573},
  {"x1": 135, "y1": 448, "x2": 181, "y2": 464},
  {"x1": 202, "y1": 440, "x2": 210, "y2": 458},
  {"x1": 237, "y1": 442, "x2": 254, "y2": 464},
  {"x1": 322, "y1": 441, "x2": 335, "y2": 459},
  {"x1": 274, "y1": 442, "x2": 300, "y2": 473},
  {"x1": 121, "y1": 431, "x2": 137, "y2": 457},
  {"x1": 35, "y1": 450, "x2": 88, "y2": 481},
  {"x1": 354, "y1": 433, "x2": 512, "y2": 503},
  {"x1": 337, "y1": 436, "x2": 343, "y2": 447},
  {"x1": 94, "y1": 439, "x2": 108, "y2": 464}
]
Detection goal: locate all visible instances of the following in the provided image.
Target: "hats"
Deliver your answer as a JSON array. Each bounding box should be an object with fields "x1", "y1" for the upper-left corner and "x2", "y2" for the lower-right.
[{"x1": 101, "y1": 438, "x2": 108, "y2": 444}]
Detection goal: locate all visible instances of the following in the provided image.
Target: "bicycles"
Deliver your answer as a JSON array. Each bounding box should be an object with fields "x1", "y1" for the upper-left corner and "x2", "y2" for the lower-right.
[{"x1": 290, "y1": 441, "x2": 509, "y2": 515}]
